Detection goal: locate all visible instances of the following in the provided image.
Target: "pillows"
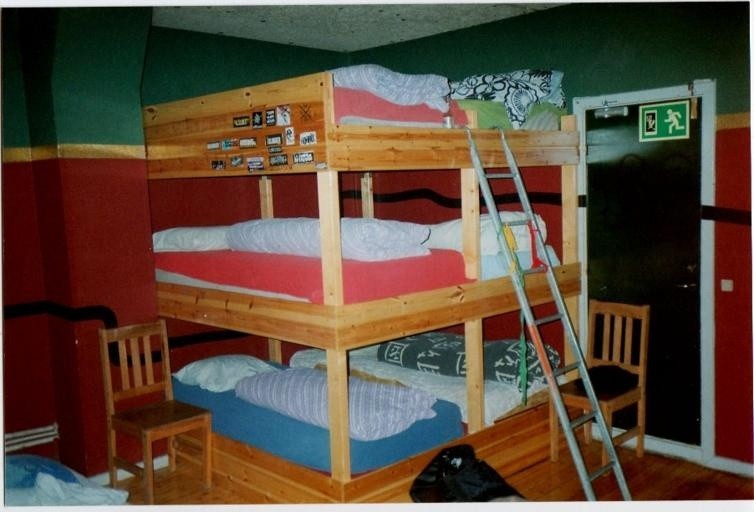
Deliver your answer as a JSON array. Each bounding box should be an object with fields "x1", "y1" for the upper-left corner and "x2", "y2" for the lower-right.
[
  {"x1": 169, "y1": 353, "x2": 282, "y2": 394},
  {"x1": 151, "y1": 225, "x2": 232, "y2": 252}
]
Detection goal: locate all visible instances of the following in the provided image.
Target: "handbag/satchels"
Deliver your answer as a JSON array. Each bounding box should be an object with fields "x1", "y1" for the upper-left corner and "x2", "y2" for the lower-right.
[
  {"x1": 409, "y1": 445, "x2": 524, "y2": 501},
  {"x1": 477, "y1": 478, "x2": 478, "y2": 489}
]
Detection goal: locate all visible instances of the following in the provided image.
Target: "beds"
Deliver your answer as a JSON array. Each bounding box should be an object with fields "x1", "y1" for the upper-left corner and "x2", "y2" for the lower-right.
[
  {"x1": 149, "y1": 212, "x2": 577, "y2": 351},
  {"x1": 146, "y1": 73, "x2": 577, "y2": 179},
  {"x1": 147, "y1": 333, "x2": 575, "y2": 501}
]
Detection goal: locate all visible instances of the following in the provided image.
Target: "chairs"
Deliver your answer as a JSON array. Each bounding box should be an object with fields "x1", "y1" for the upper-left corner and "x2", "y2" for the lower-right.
[
  {"x1": 100, "y1": 320, "x2": 213, "y2": 502},
  {"x1": 549, "y1": 298, "x2": 651, "y2": 469}
]
